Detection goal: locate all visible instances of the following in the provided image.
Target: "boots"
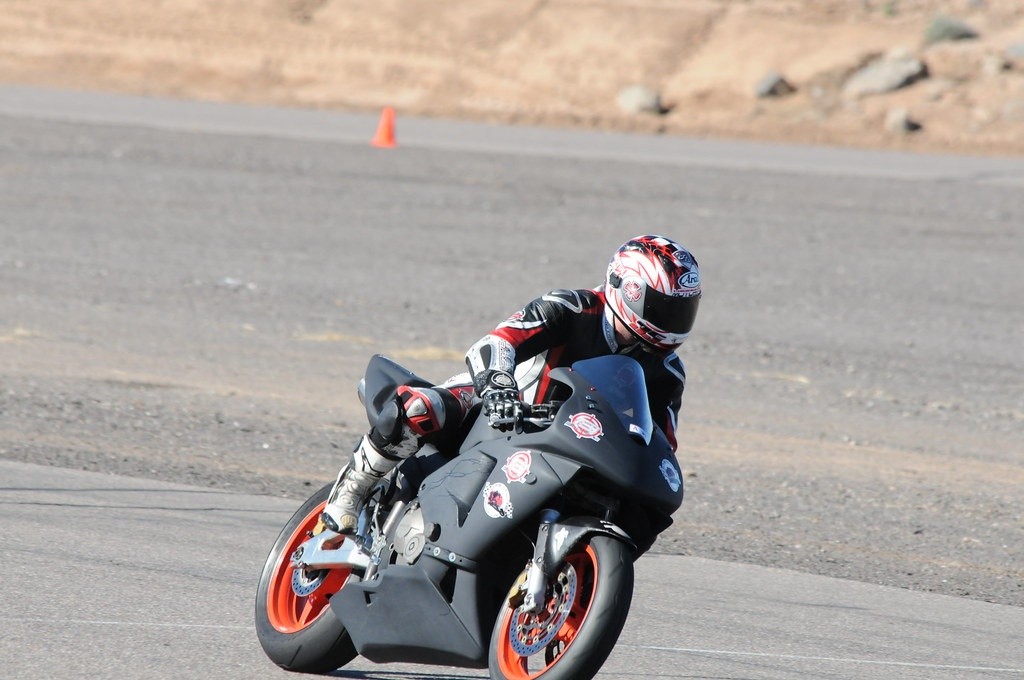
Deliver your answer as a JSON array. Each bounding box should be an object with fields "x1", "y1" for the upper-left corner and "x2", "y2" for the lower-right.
[{"x1": 321, "y1": 433, "x2": 404, "y2": 536}]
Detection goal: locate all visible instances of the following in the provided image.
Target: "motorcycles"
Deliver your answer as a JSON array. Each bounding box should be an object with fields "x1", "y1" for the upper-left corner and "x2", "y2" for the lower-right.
[{"x1": 254, "y1": 353, "x2": 682, "y2": 679}]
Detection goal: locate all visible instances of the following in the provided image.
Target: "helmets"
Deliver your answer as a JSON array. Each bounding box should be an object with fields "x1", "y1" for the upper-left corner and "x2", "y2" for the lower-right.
[{"x1": 605, "y1": 235, "x2": 702, "y2": 350}]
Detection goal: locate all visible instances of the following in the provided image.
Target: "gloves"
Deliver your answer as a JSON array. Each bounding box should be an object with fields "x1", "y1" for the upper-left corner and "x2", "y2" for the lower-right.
[{"x1": 478, "y1": 370, "x2": 524, "y2": 433}]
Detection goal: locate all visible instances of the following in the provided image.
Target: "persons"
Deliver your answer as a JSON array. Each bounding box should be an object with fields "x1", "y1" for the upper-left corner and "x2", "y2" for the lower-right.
[{"x1": 321, "y1": 234, "x2": 703, "y2": 535}]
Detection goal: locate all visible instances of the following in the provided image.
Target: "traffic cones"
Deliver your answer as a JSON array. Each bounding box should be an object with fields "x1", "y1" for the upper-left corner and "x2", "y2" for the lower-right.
[{"x1": 371, "y1": 108, "x2": 398, "y2": 149}]
